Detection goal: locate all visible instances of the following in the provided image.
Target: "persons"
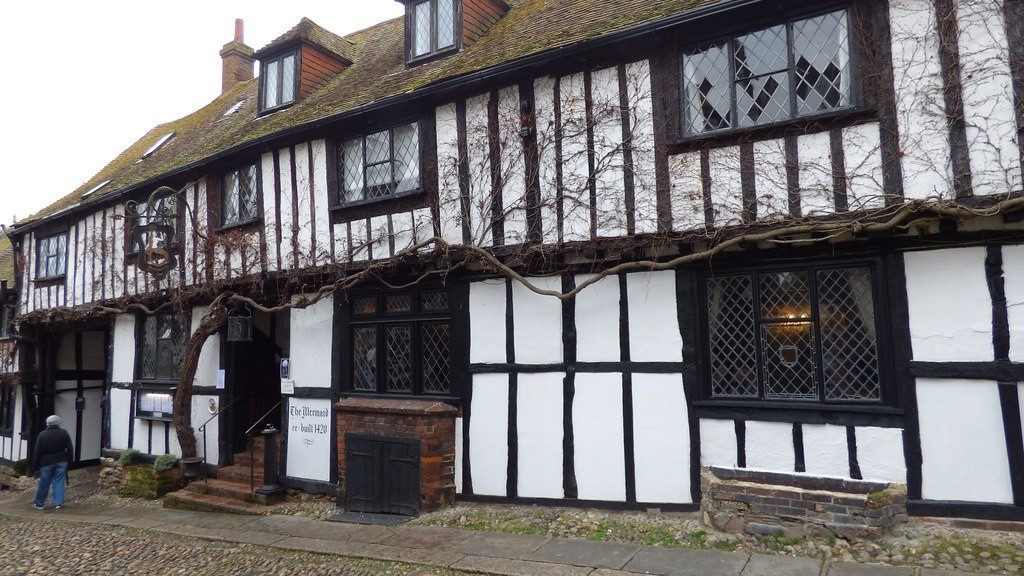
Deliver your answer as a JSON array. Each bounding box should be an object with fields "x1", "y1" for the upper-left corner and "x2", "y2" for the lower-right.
[{"x1": 32, "y1": 415, "x2": 74, "y2": 509}]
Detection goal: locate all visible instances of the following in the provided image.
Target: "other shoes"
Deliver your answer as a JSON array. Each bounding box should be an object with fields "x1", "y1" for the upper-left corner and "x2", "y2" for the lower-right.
[
  {"x1": 55, "y1": 505, "x2": 61, "y2": 509},
  {"x1": 34, "y1": 504, "x2": 44, "y2": 509}
]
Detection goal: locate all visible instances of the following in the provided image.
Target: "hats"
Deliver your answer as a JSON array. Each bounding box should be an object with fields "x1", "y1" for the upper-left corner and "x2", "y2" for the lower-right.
[{"x1": 47, "y1": 414, "x2": 61, "y2": 426}]
